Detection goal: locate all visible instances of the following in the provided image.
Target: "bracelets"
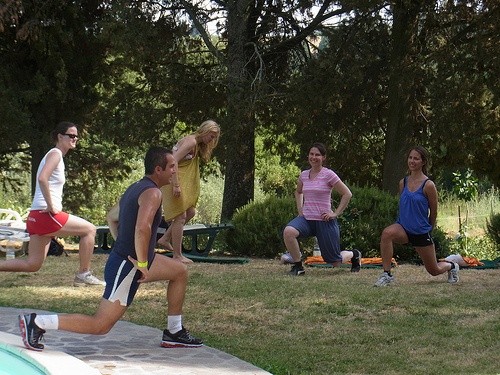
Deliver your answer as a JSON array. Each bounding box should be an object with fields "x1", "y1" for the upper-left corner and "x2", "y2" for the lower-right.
[
  {"x1": 136, "y1": 260, "x2": 149, "y2": 268},
  {"x1": 173, "y1": 184, "x2": 180, "y2": 188}
]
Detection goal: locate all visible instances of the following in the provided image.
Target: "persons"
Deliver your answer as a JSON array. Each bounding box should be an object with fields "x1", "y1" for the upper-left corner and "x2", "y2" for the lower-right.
[
  {"x1": 18, "y1": 143, "x2": 204, "y2": 352},
  {"x1": 283, "y1": 141, "x2": 361, "y2": 276},
  {"x1": 159, "y1": 119, "x2": 222, "y2": 264},
  {"x1": 0, "y1": 121, "x2": 108, "y2": 286},
  {"x1": 371, "y1": 145, "x2": 459, "y2": 289}
]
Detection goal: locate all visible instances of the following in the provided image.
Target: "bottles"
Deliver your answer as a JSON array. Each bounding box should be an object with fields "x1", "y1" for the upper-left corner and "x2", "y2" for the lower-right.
[
  {"x1": 313, "y1": 237, "x2": 321, "y2": 257},
  {"x1": 6, "y1": 237, "x2": 15, "y2": 260}
]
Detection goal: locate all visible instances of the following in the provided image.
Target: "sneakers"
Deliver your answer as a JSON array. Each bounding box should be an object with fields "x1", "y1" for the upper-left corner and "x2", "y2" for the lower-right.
[
  {"x1": 73, "y1": 271, "x2": 106, "y2": 287},
  {"x1": 447, "y1": 259, "x2": 460, "y2": 283},
  {"x1": 287, "y1": 266, "x2": 305, "y2": 276},
  {"x1": 350, "y1": 249, "x2": 361, "y2": 272},
  {"x1": 160, "y1": 325, "x2": 203, "y2": 348},
  {"x1": 372, "y1": 272, "x2": 394, "y2": 287},
  {"x1": 18, "y1": 312, "x2": 46, "y2": 350}
]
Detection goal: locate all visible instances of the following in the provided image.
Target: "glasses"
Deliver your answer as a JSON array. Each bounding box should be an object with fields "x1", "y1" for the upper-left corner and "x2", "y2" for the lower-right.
[{"x1": 63, "y1": 134, "x2": 78, "y2": 138}]
